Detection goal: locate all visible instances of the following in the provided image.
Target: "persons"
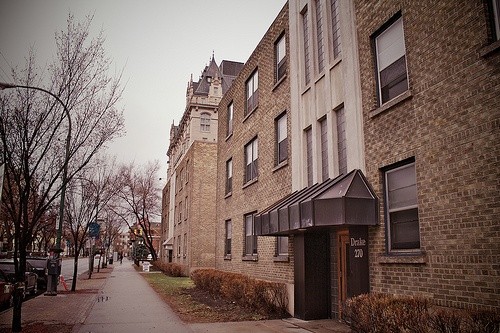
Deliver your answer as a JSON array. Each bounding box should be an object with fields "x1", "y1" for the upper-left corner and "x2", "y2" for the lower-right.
[{"x1": 120, "y1": 255, "x2": 123, "y2": 263}]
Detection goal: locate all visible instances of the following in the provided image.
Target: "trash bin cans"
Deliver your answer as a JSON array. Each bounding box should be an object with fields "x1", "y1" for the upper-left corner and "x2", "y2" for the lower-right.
[{"x1": 109, "y1": 258, "x2": 114, "y2": 264}]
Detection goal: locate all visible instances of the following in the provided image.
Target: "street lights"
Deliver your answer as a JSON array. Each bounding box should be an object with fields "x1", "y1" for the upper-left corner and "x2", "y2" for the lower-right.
[
  {"x1": 0, "y1": 81, "x2": 72, "y2": 296},
  {"x1": 63, "y1": 174, "x2": 100, "y2": 279}
]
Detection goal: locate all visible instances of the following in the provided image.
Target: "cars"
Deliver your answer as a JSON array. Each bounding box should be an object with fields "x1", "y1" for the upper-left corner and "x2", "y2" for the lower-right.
[{"x1": 0, "y1": 257, "x2": 61, "y2": 307}]
